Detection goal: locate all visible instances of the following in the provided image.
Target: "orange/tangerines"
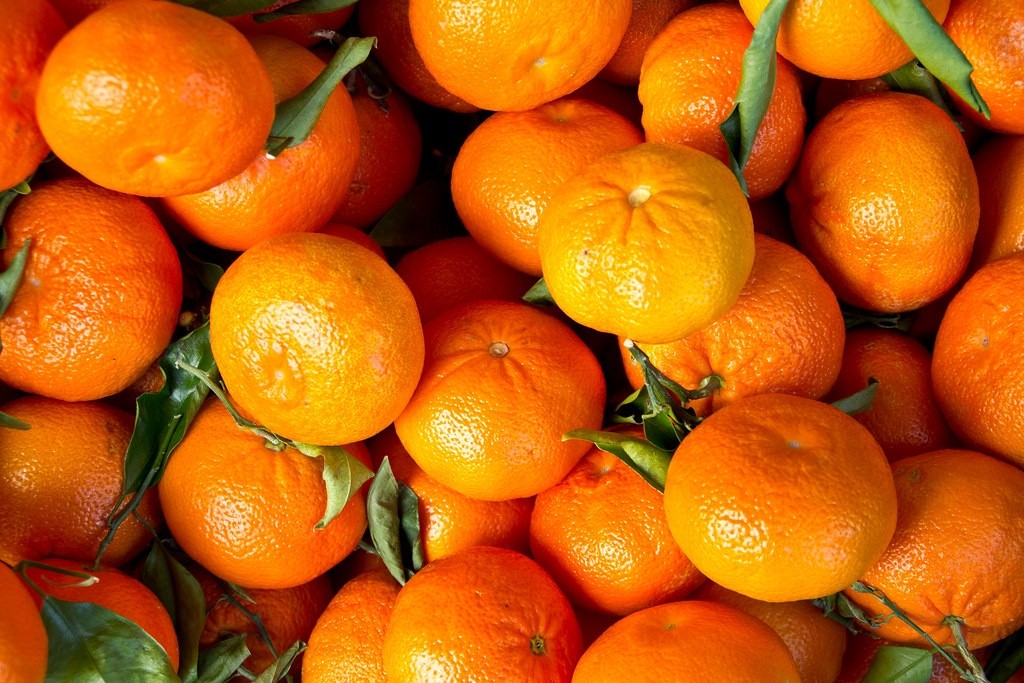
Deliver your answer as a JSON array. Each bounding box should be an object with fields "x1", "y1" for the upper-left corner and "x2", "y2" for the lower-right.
[{"x1": 0, "y1": 0, "x2": 1024, "y2": 683}]
